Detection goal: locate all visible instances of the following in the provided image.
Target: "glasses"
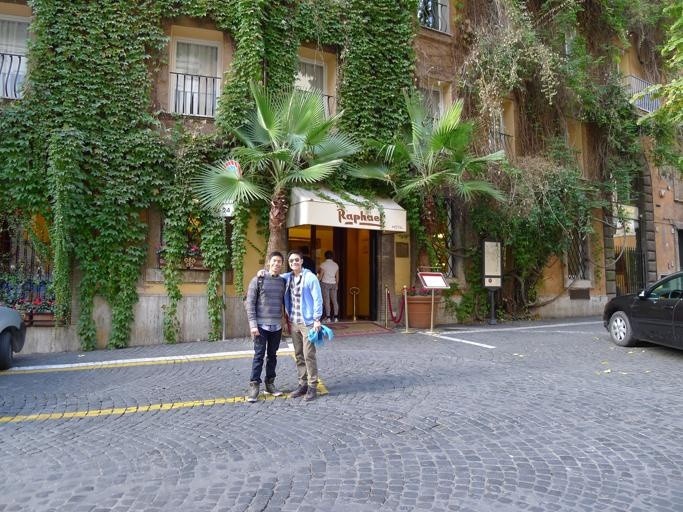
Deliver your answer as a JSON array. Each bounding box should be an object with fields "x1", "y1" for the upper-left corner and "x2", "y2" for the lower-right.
[{"x1": 290, "y1": 258, "x2": 299, "y2": 262}]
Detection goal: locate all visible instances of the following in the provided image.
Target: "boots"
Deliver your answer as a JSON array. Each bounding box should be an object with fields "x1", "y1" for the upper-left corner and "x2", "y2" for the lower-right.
[
  {"x1": 264, "y1": 377, "x2": 283, "y2": 396},
  {"x1": 248, "y1": 381, "x2": 259, "y2": 402}
]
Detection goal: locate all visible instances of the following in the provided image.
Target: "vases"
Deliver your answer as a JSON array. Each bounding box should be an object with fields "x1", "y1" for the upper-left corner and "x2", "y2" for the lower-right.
[{"x1": 401, "y1": 294, "x2": 440, "y2": 330}]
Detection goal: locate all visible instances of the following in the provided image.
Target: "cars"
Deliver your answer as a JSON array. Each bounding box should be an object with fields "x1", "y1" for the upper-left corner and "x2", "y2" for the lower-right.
[
  {"x1": 603, "y1": 269, "x2": 683, "y2": 353},
  {"x1": 0, "y1": 307, "x2": 29, "y2": 369}
]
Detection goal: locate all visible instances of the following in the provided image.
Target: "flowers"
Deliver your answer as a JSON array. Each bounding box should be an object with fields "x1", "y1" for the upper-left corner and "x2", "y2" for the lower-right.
[{"x1": 401, "y1": 284, "x2": 439, "y2": 297}]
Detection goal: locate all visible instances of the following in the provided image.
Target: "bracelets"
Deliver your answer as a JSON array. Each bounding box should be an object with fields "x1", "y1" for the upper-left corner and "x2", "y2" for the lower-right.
[{"x1": 313, "y1": 318, "x2": 320, "y2": 322}]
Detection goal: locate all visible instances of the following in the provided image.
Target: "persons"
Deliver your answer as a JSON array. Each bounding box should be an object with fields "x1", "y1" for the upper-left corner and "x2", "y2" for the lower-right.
[
  {"x1": 317, "y1": 250, "x2": 341, "y2": 323},
  {"x1": 256, "y1": 249, "x2": 324, "y2": 402},
  {"x1": 245, "y1": 251, "x2": 287, "y2": 403}
]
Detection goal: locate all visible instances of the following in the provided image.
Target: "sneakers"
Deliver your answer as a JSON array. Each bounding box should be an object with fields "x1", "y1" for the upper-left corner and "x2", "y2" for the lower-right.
[{"x1": 291, "y1": 384, "x2": 316, "y2": 401}]
